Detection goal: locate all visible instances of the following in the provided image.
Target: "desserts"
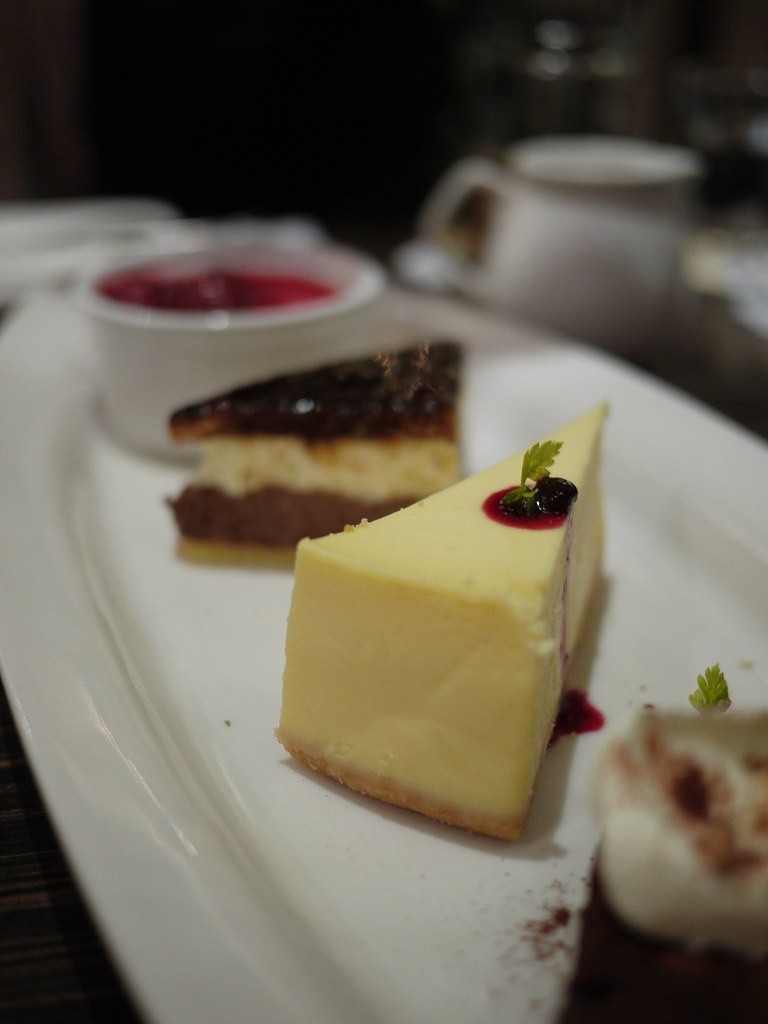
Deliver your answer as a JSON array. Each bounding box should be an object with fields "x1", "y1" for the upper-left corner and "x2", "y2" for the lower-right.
[
  {"x1": 275, "y1": 403, "x2": 608, "y2": 843},
  {"x1": 556, "y1": 667, "x2": 768, "y2": 1024},
  {"x1": 162, "y1": 335, "x2": 471, "y2": 567}
]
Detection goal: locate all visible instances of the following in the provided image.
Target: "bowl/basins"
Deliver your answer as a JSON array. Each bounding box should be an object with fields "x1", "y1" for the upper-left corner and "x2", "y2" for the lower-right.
[{"x1": 81, "y1": 240, "x2": 393, "y2": 464}]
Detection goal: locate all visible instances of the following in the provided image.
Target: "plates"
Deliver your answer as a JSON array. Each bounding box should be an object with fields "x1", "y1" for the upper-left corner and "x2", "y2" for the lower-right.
[{"x1": 1, "y1": 198, "x2": 768, "y2": 1024}]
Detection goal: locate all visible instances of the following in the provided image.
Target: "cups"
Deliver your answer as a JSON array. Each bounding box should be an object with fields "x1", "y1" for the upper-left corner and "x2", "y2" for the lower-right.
[{"x1": 420, "y1": 133, "x2": 703, "y2": 364}]
